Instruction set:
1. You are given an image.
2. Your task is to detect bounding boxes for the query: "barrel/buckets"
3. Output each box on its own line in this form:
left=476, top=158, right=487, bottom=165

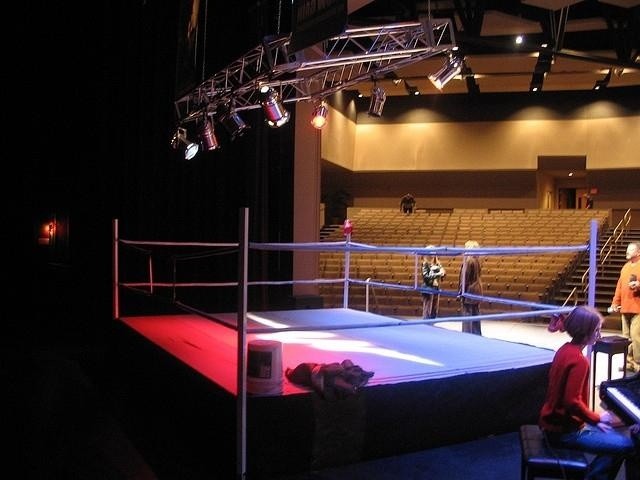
left=248, top=341, right=283, bottom=397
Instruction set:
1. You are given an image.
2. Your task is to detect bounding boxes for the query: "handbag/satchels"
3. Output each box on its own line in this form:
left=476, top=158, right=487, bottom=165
left=464, top=280, right=484, bottom=305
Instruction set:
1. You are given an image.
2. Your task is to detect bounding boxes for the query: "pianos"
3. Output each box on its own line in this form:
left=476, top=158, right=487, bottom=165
left=600, top=369, right=639, bottom=479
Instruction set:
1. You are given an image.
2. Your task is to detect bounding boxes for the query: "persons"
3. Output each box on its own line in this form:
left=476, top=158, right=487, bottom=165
left=455, top=239, right=484, bottom=337
left=536, top=305, right=635, bottom=480
left=605, top=241, right=640, bottom=374
left=399, top=193, right=417, bottom=216
left=421, top=245, right=446, bottom=320
left=583, top=193, right=594, bottom=209
